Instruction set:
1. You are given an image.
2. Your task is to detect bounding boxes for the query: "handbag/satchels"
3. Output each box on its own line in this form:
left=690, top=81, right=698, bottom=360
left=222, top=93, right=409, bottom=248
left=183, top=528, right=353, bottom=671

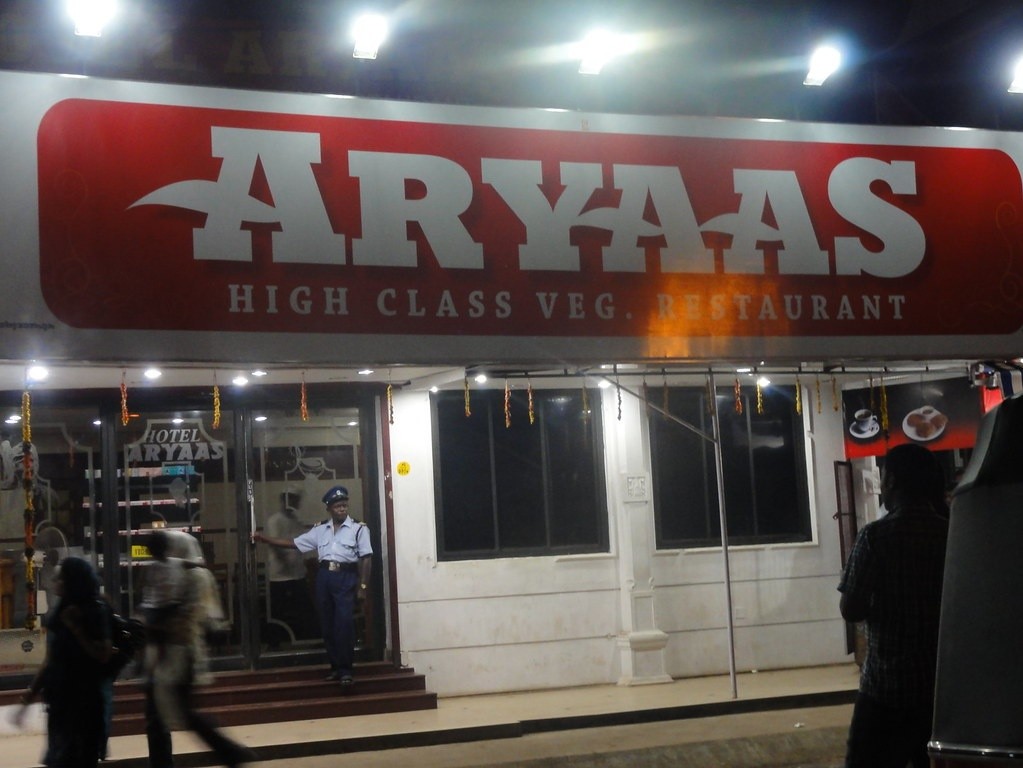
left=80, top=601, right=145, bottom=684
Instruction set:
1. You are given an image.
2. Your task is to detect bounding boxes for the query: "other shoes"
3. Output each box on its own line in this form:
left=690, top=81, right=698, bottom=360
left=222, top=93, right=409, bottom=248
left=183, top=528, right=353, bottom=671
left=326, top=673, right=338, bottom=681
left=341, top=675, right=352, bottom=684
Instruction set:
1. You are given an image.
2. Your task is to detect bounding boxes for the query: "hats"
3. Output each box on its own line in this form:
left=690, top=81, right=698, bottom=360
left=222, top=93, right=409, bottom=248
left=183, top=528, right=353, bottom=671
left=322, top=486, right=349, bottom=506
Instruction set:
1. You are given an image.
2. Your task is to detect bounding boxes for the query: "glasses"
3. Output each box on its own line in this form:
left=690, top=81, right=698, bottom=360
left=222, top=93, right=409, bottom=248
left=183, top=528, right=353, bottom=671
left=946, top=481, right=959, bottom=491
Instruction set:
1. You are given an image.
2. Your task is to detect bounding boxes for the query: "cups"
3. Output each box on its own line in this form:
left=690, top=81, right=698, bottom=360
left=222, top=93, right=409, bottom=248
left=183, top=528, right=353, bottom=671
left=855, top=410, right=876, bottom=431
left=921, top=406, right=935, bottom=419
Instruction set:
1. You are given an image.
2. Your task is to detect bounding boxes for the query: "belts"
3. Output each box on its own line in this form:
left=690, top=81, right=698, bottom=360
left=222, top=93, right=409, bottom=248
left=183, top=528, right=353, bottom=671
left=321, top=560, right=358, bottom=572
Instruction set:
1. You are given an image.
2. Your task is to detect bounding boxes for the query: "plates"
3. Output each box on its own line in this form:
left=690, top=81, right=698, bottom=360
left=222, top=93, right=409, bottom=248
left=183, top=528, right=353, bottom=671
left=902, top=408, right=944, bottom=440
left=849, top=422, right=879, bottom=439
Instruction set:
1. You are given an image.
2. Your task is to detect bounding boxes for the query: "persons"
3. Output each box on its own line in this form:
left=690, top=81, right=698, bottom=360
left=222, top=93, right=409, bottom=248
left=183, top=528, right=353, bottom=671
left=249, top=486, right=374, bottom=686
left=836, top=443, right=950, bottom=768
left=11, top=556, right=118, bottom=768
left=265, top=487, right=310, bottom=644
left=132, top=530, right=259, bottom=768
